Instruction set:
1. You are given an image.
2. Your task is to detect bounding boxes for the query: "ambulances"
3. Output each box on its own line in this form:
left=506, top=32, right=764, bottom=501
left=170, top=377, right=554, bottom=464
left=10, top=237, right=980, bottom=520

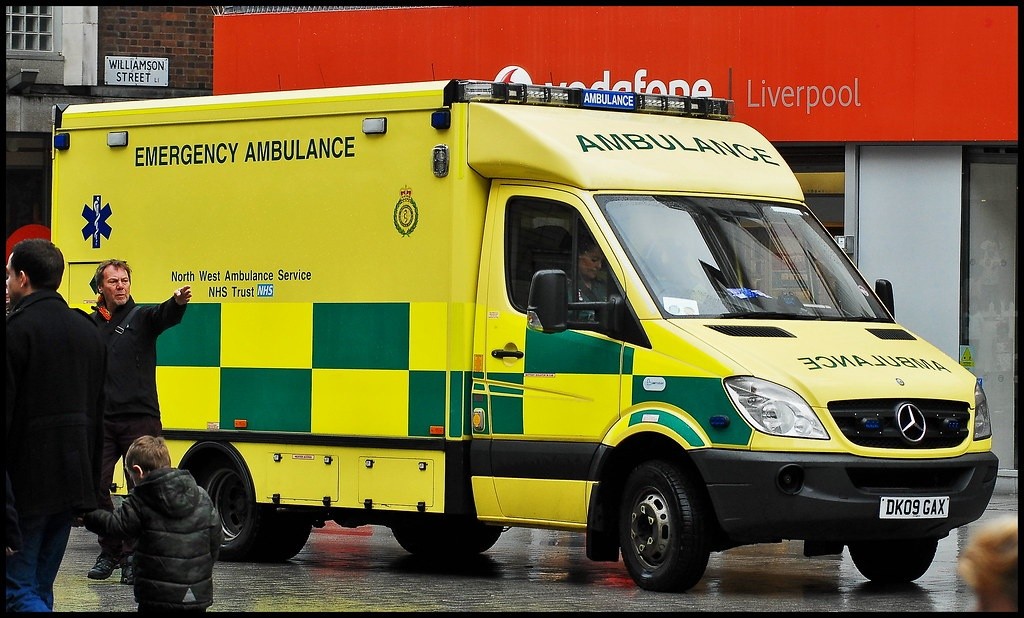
left=48, top=65, right=1001, bottom=594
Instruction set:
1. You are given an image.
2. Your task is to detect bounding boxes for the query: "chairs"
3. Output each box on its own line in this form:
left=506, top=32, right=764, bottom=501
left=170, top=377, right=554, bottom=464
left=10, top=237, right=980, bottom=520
left=517, top=225, right=572, bottom=282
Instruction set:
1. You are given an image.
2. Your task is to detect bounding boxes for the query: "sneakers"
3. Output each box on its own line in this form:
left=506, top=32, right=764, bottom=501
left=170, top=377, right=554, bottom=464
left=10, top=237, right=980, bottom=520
left=121, top=556, right=134, bottom=585
left=87, top=550, right=121, bottom=580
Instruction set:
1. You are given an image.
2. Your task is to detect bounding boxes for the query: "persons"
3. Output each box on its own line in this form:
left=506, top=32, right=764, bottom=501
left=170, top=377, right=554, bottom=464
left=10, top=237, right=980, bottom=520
left=958, top=515, right=1018, bottom=612
left=6, top=238, right=108, bottom=613
left=559, top=226, right=606, bottom=323
left=88, top=261, right=192, bottom=585
left=6, top=472, right=23, bottom=556
left=84, top=435, right=222, bottom=613
